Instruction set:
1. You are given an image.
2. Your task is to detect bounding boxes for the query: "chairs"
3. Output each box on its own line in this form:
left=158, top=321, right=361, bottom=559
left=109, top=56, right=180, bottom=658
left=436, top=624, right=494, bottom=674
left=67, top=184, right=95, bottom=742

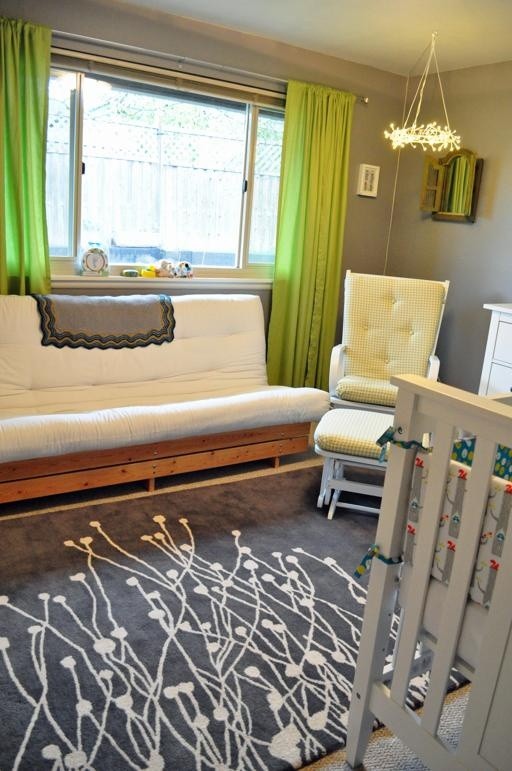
left=328, top=268, right=450, bottom=413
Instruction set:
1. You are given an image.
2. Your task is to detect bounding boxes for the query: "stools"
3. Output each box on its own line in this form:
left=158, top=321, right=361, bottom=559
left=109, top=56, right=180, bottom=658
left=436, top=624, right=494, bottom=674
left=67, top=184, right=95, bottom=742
left=312, top=408, right=430, bottom=521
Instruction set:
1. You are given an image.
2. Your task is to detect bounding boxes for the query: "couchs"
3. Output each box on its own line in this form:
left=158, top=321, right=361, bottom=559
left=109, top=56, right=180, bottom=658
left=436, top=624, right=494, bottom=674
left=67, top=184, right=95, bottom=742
left=1, top=293, right=331, bottom=507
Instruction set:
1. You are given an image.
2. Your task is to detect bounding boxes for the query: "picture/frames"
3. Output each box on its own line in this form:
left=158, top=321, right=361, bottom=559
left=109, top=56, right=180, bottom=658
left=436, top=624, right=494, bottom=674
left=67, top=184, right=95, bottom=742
left=356, top=162, right=380, bottom=197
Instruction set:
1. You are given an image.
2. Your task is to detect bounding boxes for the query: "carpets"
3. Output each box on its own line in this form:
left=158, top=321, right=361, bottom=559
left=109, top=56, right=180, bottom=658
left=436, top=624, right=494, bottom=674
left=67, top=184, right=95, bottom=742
left=0, top=461, right=472, bottom=770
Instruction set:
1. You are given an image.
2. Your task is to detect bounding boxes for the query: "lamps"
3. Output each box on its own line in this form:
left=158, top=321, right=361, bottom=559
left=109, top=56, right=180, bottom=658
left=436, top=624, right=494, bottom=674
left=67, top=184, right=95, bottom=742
left=383, top=29, right=464, bottom=154
